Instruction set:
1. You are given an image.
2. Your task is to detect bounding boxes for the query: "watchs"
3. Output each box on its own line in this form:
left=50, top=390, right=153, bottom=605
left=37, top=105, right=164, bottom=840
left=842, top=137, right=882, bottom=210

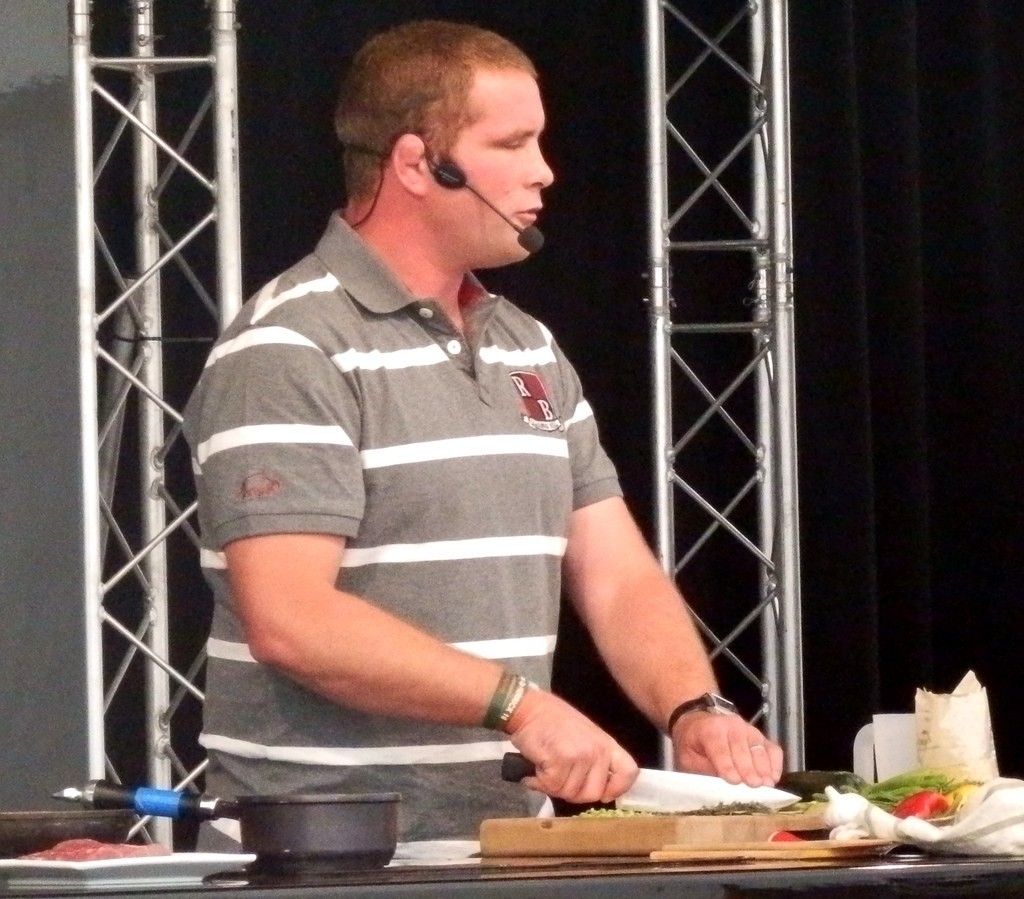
left=667, top=694, right=739, bottom=736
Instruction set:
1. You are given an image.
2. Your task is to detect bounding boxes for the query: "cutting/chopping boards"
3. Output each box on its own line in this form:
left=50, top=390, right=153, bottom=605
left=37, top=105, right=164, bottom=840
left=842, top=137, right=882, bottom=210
left=478, top=810, right=839, bottom=857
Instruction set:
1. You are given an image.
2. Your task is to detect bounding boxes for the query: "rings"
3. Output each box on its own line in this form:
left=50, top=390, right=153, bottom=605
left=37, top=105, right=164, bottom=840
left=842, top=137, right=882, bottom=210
left=750, top=744, right=764, bottom=750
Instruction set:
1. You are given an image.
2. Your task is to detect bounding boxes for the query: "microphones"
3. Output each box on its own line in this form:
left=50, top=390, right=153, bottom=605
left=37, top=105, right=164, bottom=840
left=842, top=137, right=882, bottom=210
left=432, top=163, right=545, bottom=253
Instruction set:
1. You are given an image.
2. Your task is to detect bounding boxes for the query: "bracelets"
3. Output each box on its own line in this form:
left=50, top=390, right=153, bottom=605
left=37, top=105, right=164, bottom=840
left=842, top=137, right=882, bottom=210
left=483, top=671, right=539, bottom=733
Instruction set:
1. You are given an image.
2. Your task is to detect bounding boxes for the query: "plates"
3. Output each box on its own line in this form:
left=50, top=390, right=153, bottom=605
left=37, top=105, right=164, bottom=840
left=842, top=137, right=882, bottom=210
left=0, top=852, right=258, bottom=892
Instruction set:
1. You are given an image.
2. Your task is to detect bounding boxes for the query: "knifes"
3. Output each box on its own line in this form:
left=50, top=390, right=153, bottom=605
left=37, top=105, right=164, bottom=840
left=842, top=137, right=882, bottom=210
left=501, top=753, right=802, bottom=809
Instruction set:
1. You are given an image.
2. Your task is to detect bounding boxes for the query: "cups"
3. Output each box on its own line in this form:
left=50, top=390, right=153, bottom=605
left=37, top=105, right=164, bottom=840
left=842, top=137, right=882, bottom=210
left=851, top=713, right=918, bottom=786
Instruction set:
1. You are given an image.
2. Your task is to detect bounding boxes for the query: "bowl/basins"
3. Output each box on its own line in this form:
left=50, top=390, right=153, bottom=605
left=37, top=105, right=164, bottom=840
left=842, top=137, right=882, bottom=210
left=0, top=809, right=139, bottom=855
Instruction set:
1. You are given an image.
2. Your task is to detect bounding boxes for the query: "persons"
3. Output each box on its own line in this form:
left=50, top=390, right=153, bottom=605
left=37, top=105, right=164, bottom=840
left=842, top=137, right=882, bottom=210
left=182, top=21, right=786, bottom=853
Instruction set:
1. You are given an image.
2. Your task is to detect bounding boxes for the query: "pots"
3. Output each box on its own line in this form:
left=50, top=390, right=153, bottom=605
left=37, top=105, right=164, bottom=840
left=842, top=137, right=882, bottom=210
left=49, top=779, right=403, bottom=872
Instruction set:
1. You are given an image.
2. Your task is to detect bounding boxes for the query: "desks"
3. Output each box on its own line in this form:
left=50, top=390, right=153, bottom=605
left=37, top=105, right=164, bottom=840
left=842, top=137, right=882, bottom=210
left=0, top=850, right=1024, bottom=899
